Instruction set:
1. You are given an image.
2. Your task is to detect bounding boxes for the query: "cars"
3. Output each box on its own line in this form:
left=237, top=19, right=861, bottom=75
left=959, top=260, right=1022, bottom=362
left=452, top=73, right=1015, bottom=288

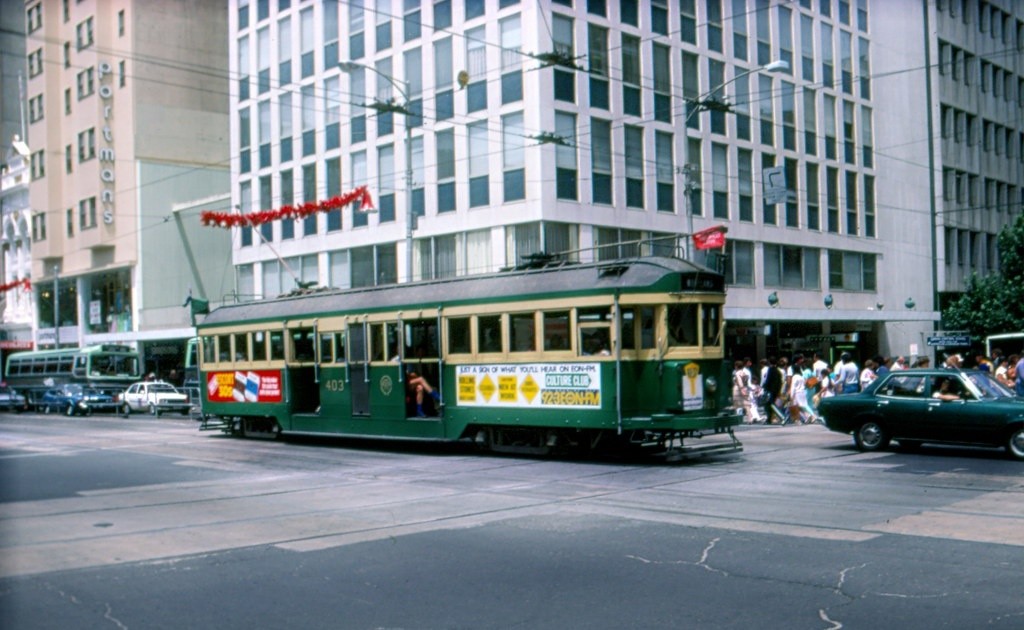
left=115, top=382, right=192, bottom=416
left=0, top=383, right=26, bottom=414
left=34, top=383, right=117, bottom=414
left=817, top=368, right=1024, bottom=463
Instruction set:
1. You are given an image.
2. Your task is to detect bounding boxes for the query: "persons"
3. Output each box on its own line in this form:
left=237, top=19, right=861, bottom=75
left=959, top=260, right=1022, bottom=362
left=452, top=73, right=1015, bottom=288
left=732, top=348, right=1024, bottom=425
left=485, top=329, right=502, bottom=352
left=391, top=345, right=440, bottom=418
left=582, top=332, right=610, bottom=356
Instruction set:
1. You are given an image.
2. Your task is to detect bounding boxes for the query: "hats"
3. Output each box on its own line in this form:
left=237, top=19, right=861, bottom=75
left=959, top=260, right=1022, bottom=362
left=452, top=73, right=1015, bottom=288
left=991, top=349, right=1002, bottom=356
left=917, top=356, right=929, bottom=366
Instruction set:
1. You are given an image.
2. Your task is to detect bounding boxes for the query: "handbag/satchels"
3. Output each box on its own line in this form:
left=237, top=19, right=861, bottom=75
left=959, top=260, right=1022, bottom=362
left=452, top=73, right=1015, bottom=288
left=787, top=399, right=799, bottom=421
left=734, top=375, right=749, bottom=387
left=807, top=376, right=818, bottom=389
left=757, top=388, right=770, bottom=407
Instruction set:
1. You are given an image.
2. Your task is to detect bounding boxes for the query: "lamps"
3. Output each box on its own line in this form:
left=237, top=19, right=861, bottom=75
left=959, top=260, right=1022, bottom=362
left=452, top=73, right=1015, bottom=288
left=768, top=292, right=778, bottom=308
left=906, top=297, right=915, bottom=311
left=824, top=294, right=833, bottom=309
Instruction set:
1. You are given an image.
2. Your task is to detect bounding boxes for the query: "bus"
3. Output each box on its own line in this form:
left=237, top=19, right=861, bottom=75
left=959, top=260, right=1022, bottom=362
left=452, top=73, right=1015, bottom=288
left=184, top=337, right=207, bottom=399
left=4, top=344, right=142, bottom=397
left=181, top=224, right=745, bottom=463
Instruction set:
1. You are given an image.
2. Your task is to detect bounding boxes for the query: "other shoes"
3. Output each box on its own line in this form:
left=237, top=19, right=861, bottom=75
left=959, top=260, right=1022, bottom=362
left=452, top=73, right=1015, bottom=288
left=804, top=413, right=811, bottom=424
left=811, top=415, right=818, bottom=425
left=763, top=419, right=771, bottom=425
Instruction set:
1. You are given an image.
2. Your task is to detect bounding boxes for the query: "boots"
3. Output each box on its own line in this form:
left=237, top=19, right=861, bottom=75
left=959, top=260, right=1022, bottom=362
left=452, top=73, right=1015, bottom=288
left=429, top=391, right=440, bottom=402
left=417, top=404, right=426, bottom=418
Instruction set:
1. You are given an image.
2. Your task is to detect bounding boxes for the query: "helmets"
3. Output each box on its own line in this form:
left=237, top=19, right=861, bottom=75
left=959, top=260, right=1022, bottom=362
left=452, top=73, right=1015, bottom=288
left=841, top=351, right=852, bottom=363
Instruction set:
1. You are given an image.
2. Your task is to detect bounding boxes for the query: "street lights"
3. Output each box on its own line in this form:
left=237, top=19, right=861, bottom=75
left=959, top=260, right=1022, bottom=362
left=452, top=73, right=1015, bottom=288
left=336, top=60, right=413, bottom=284
left=683, top=60, right=790, bottom=265
